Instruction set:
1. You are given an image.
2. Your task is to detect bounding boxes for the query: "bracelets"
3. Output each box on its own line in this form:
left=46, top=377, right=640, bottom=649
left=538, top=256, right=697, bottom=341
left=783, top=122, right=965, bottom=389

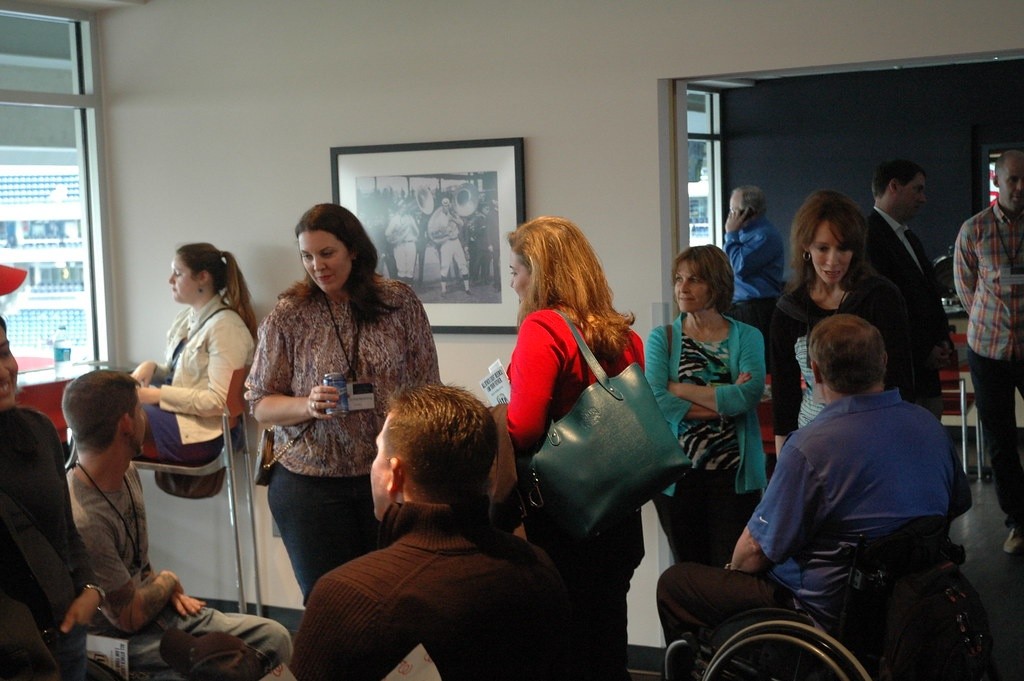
left=85, top=584, right=106, bottom=607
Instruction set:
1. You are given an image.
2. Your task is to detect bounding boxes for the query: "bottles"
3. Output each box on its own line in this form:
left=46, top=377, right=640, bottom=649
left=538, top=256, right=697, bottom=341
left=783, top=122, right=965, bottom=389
left=54, top=324, right=71, bottom=380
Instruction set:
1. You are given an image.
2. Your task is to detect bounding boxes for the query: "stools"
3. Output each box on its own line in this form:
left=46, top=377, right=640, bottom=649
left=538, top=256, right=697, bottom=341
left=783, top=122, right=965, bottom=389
left=133, top=363, right=267, bottom=615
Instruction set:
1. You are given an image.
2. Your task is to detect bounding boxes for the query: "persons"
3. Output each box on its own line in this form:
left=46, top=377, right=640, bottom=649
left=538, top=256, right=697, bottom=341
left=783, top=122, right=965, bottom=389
left=953, top=150, right=1024, bottom=553
left=644, top=160, right=955, bottom=566
left=499, top=215, right=692, bottom=681
left=245, top=204, right=440, bottom=605
left=0, top=312, right=123, bottom=681
left=281, top=384, right=568, bottom=681
left=61, top=370, right=294, bottom=680
left=655, top=316, right=1000, bottom=681
left=125, top=243, right=262, bottom=464
left=355, top=175, right=500, bottom=299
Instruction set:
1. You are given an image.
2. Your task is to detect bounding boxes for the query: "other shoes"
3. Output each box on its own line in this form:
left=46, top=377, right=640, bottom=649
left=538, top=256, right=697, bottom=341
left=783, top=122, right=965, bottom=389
left=1004, top=527, right=1024, bottom=554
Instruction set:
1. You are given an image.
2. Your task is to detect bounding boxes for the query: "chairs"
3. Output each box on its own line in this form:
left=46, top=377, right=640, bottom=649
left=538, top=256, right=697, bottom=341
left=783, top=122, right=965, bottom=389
left=938, top=349, right=981, bottom=485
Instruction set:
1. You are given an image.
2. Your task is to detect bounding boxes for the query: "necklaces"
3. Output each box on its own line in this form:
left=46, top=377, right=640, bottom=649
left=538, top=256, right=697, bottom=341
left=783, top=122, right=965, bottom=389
left=683, top=320, right=724, bottom=348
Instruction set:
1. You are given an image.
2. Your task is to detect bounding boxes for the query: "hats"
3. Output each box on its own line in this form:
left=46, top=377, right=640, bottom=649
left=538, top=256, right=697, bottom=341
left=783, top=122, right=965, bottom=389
left=160, top=628, right=264, bottom=681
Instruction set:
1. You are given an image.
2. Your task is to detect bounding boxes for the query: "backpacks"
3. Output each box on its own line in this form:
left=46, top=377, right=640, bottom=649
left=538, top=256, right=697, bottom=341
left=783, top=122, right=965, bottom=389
left=878, top=562, right=994, bottom=681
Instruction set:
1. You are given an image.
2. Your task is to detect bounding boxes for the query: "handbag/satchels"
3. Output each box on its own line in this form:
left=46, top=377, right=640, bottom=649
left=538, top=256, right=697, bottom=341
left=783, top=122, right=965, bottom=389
left=153, top=464, right=226, bottom=500
left=516, top=309, right=692, bottom=543
left=253, top=428, right=275, bottom=485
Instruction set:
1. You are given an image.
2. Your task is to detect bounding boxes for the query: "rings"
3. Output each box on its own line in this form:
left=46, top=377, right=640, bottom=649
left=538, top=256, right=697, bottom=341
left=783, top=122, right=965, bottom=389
left=311, top=402, right=316, bottom=409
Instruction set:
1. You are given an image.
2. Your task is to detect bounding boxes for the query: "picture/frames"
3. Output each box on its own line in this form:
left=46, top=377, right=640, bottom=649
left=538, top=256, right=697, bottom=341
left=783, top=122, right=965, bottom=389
left=329, top=138, right=531, bottom=335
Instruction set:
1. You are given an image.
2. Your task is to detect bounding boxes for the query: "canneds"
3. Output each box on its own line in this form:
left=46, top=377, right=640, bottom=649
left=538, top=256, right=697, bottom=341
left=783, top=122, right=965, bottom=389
left=323, top=372, right=349, bottom=416
